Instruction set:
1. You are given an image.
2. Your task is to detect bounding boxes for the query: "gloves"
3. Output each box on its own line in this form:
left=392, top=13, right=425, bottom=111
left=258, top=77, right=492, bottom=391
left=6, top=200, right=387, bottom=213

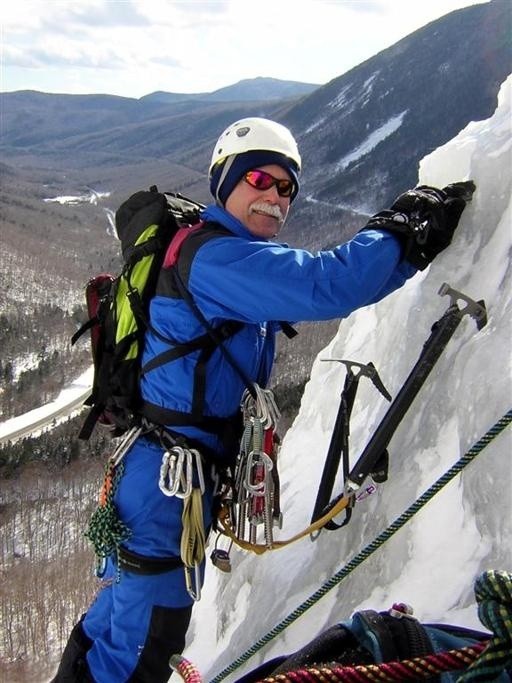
left=358, top=179, right=476, bottom=271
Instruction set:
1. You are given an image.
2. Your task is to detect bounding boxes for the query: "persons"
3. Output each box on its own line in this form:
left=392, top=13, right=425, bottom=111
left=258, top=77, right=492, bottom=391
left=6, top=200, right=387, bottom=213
left=52, top=118, right=472, bottom=682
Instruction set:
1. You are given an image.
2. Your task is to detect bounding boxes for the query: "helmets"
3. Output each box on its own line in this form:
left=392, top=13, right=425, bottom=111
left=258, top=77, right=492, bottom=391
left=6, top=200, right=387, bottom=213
left=208, top=117, right=302, bottom=178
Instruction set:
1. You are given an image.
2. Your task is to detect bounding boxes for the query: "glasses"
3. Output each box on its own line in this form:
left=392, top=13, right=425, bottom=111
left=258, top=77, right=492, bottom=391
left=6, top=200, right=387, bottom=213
left=239, top=166, right=294, bottom=199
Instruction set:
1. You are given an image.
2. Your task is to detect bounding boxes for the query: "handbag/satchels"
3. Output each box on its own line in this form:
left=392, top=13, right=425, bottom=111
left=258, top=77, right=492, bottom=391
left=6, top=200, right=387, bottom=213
left=259, top=602, right=490, bottom=683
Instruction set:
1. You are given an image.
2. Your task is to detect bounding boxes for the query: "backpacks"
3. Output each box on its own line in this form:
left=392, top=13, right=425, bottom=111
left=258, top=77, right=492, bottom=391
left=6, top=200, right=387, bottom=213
left=85, top=184, right=238, bottom=429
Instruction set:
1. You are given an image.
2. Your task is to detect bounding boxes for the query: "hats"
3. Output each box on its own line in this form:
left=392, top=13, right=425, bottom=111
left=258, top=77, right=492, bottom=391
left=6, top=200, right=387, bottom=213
left=209, top=151, right=300, bottom=210
